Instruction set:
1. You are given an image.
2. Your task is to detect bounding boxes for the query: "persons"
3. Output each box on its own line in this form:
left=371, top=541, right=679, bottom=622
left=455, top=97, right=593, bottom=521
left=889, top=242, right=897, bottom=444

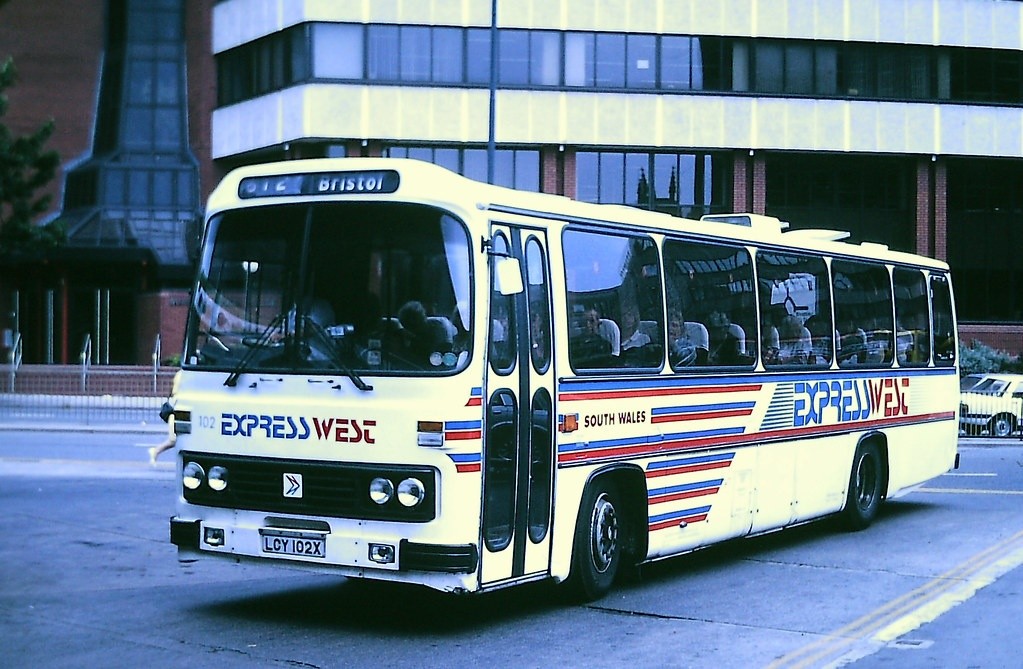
left=612, top=303, right=652, bottom=352
left=569, top=305, right=614, bottom=368
left=702, top=311, right=909, bottom=366
left=269, top=279, right=337, bottom=359
left=667, top=308, right=697, bottom=367
left=396, top=300, right=447, bottom=355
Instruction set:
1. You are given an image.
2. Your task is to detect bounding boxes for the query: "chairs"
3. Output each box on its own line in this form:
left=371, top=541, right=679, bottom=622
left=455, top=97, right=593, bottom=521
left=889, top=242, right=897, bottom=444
left=368, top=308, right=938, bottom=375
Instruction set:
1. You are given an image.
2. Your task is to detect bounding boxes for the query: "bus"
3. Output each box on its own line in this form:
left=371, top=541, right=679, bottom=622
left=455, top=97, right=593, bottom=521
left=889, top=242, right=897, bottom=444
left=168, top=158, right=962, bottom=602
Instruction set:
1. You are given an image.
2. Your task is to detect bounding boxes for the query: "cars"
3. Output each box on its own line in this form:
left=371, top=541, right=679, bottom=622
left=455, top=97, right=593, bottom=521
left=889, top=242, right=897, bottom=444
left=962, top=373, right=1023, bottom=437
